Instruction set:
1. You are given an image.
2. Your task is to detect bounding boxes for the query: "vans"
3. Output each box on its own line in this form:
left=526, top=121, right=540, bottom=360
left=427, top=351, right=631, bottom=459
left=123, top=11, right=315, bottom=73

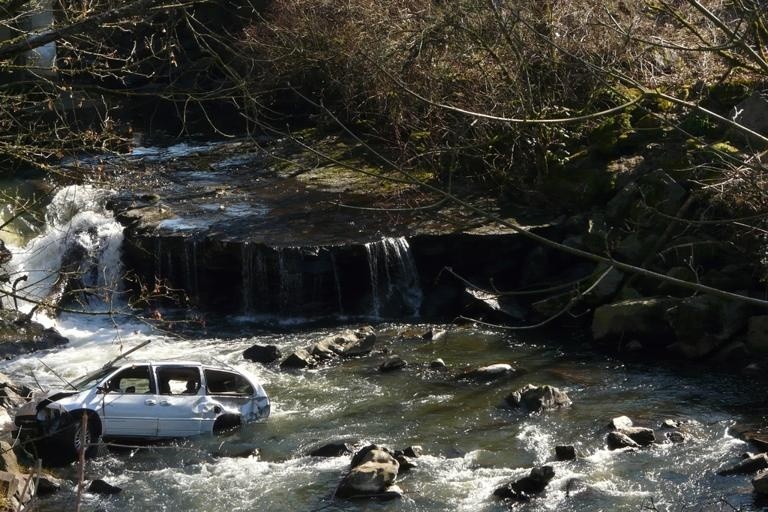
left=16, top=361, right=272, bottom=459
